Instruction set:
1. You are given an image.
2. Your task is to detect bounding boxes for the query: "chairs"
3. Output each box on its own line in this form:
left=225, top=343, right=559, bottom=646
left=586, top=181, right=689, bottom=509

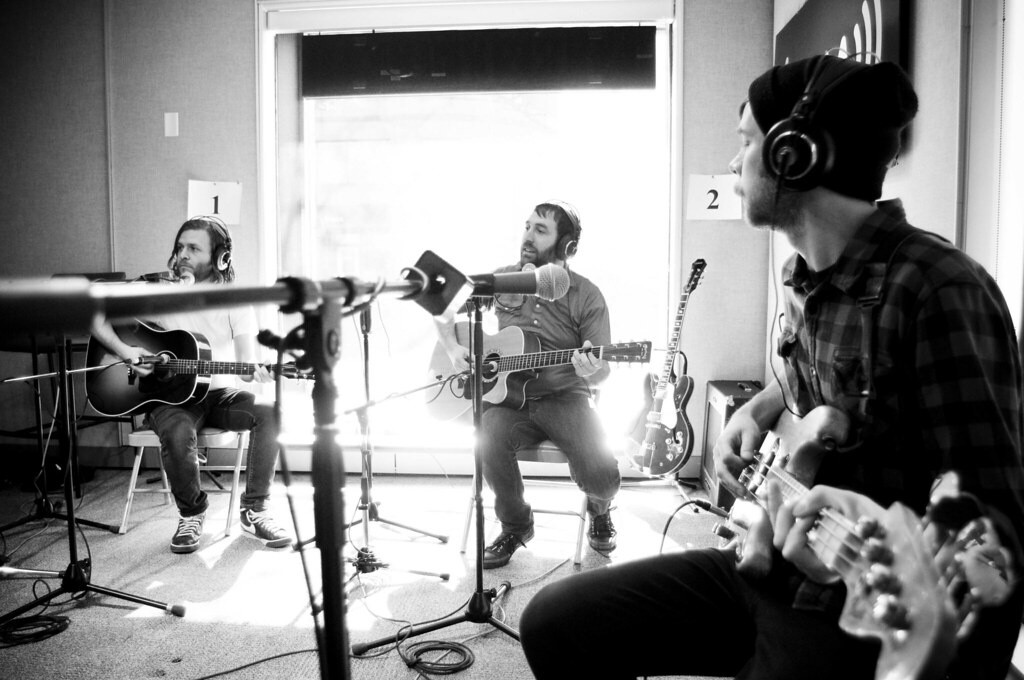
left=118, top=423, right=249, bottom=537
left=457, top=388, right=601, bottom=565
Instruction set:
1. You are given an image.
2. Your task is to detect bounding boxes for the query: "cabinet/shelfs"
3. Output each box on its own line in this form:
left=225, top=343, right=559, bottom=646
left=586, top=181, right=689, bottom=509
left=0, top=339, right=142, bottom=499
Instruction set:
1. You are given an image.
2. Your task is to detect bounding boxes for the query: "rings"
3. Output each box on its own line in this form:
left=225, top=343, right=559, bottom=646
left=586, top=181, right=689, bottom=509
left=580, top=364, right=583, bottom=367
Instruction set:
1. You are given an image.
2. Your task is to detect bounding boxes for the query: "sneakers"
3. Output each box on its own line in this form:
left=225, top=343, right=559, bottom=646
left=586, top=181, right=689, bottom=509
left=587, top=506, right=618, bottom=550
left=483, top=525, right=535, bottom=569
left=239, top=506, right=292, bottom=548
left=171, top=511, right=206, bottom=552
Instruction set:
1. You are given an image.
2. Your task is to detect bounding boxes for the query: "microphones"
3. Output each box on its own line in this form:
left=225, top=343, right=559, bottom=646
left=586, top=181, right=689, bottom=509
left=463, top=262, right=570, bottom=302
left=462, top=360, right=497, bottom=375
left=178, top=272, right=195, bottom=286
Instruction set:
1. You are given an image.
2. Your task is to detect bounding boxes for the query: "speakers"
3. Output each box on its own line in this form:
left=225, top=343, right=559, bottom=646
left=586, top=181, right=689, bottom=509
left=701, top=381, right=768, bottom=508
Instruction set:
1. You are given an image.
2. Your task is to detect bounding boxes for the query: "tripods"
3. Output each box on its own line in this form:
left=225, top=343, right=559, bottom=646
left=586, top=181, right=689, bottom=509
left=0, top=332, right=186, bottom=623
left=293, top=278, right=523, bottom=658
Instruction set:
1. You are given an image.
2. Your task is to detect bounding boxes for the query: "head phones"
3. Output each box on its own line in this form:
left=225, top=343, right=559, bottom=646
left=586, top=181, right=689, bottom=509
left=763, top=48, right=881, bottom=191
left=545, top=201, right=580, bottom=261
left=187, top=215, right=232, bottom=271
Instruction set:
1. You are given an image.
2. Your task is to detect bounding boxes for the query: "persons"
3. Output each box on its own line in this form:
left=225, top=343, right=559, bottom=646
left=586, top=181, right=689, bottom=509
left=89, top=219, right=292, bottom=556
left=519, top=53, right=1024, bottom=680
left=434, top=202, right=623, bottom=570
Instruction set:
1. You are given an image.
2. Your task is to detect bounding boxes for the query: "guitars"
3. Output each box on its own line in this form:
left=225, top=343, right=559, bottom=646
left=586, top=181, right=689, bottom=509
left=623, top=258, right=707, bottom=479
left=83, top=317, right=315, bottom=417
left=713, top=404, right=1014, bottom=680
left=425, top=322, right=652, bottom=426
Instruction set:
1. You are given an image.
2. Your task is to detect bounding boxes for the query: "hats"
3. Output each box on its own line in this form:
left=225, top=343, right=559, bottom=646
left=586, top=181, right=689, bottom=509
left=749, top=54, right=919, bottom=202
